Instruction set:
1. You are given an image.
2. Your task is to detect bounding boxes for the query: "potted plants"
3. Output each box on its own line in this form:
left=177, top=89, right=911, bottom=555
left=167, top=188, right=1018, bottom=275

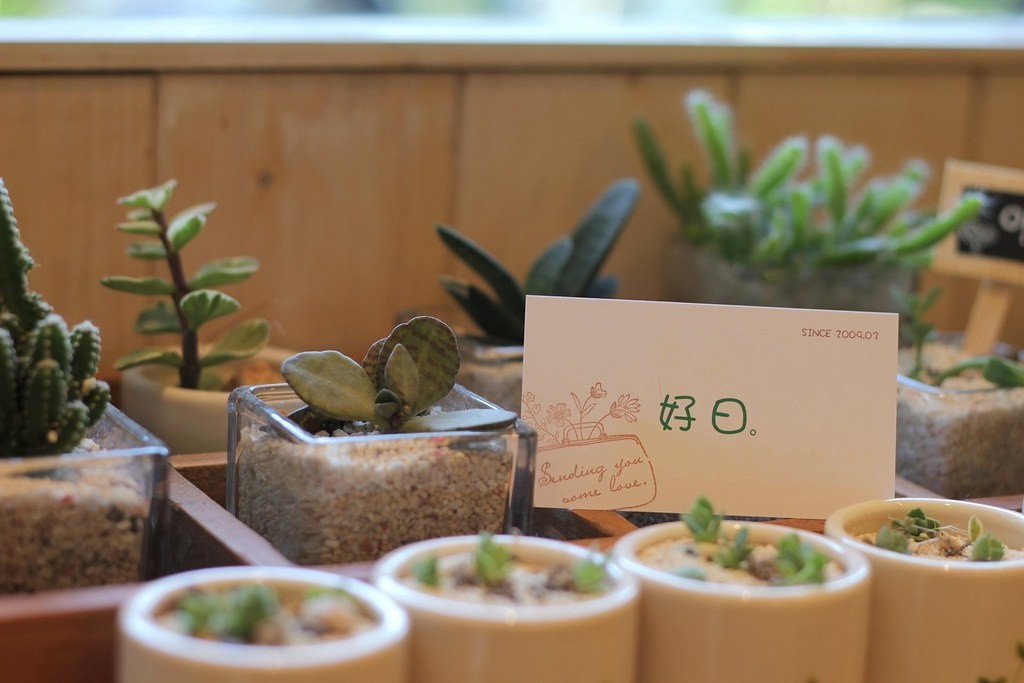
left=0, top=86, right=1024, bottom=682
